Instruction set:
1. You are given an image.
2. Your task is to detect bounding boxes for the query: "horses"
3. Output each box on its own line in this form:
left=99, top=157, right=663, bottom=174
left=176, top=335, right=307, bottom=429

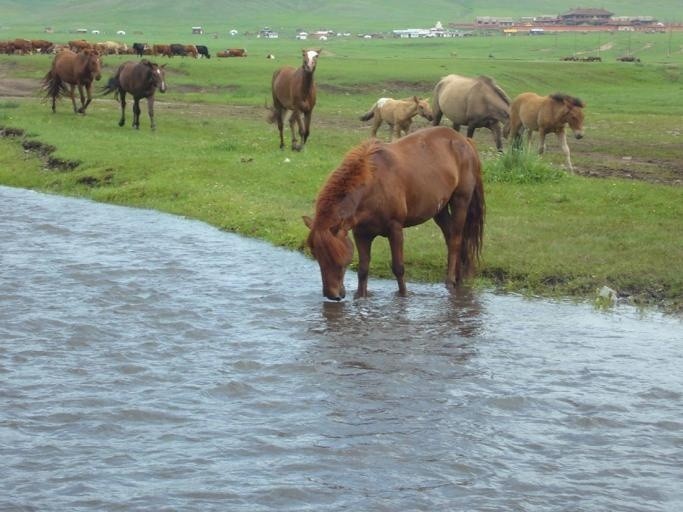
left=99, top=59, right=167, bottom=131
left=40, top=49, right=102, bottom=114
left=359, top=95, right=433, bottom=140
left=301, top=125, right=487, bottom=301
left=217, top=48, right=248, bottom=57
left=265, top=48, right=323, bottom=152
left=432, top=74, right=524, bottom=155
left=0, top=39, right=213, bottom=56
left=509, top=92, right=585, bottom=173
